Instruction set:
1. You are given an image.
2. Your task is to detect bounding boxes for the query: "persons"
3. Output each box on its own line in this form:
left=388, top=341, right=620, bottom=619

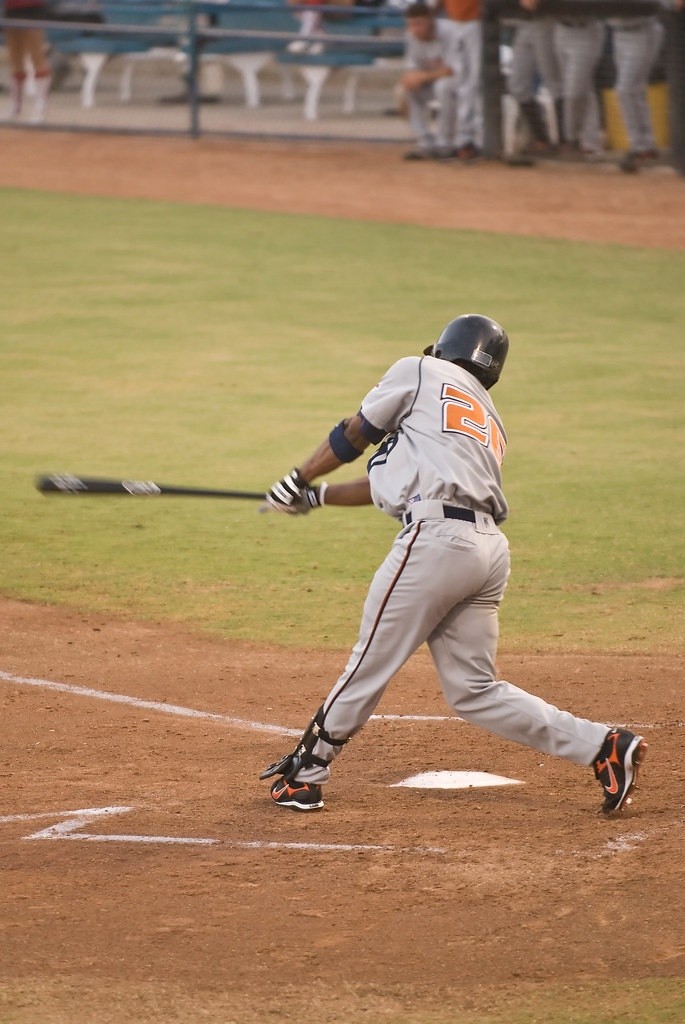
left=158, top=0, right=229, bottom=104
left=265, top=312, right=649, bottom=816
left=285, top=0, right=354, bottom=54
left=4, top=0, right=55, bottom=121
left=50, top=0, right=107, bottom=80
left=381, top=0, right=685, bottom=175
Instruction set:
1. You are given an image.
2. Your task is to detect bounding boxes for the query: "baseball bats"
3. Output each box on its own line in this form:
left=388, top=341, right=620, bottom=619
left=34, top=471, right=269, bottom=501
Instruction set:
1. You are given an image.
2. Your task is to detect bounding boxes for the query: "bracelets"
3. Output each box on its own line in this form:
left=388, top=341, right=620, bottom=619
left=319, top=482, right=327, bottom=507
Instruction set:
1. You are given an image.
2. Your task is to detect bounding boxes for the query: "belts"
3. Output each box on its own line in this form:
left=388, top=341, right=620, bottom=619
left=405, top=504, right=476, bottom=523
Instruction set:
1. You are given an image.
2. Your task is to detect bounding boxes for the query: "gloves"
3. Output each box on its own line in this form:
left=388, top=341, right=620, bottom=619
left=264, top=468, right=327, bottom=515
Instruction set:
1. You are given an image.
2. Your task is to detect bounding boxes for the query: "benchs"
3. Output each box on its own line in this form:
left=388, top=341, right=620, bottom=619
left=0, top=0, right=618, bottom=145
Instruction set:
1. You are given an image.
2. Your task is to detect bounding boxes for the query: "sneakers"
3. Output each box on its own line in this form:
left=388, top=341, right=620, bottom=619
left=593, top=728, right=647, bottom=815
left=270, top=776, right=324, bottom=812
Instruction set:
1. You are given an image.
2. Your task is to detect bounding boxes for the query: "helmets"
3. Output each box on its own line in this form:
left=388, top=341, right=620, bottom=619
left=423, top=314, right=509, bottom=390
